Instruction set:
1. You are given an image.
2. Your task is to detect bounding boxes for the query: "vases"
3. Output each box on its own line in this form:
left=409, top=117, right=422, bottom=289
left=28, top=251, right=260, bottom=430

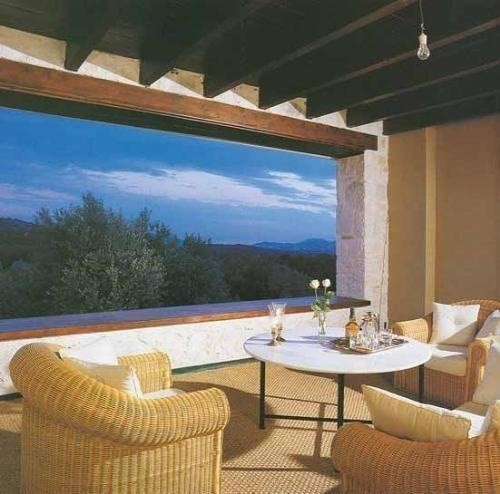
left=309, top=278, right=335, bottom=335
left=316, top=309, right=329, bottom=337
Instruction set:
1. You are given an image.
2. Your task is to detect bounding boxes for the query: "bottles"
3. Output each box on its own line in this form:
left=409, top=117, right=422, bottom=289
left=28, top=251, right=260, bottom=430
left=347, top=305, right=360, bottom=343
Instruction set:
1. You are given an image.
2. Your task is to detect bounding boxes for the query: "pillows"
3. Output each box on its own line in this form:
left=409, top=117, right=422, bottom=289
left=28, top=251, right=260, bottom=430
left=56, top=335, right=142, bottom=397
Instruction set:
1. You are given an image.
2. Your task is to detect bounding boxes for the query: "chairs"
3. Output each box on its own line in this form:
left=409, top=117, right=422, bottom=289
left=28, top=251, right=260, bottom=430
left=7, top=339, right=234, bottom=494
left=328, top=298, right=500, bottom=494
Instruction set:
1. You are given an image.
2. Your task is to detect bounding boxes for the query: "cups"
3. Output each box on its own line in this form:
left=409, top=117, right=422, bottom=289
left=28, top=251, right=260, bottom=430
left=350, top=322, right=392, bottom=352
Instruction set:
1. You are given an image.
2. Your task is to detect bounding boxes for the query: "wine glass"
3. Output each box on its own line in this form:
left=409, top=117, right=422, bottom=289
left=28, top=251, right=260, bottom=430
left=268, top=302, right=286, bottom=346
